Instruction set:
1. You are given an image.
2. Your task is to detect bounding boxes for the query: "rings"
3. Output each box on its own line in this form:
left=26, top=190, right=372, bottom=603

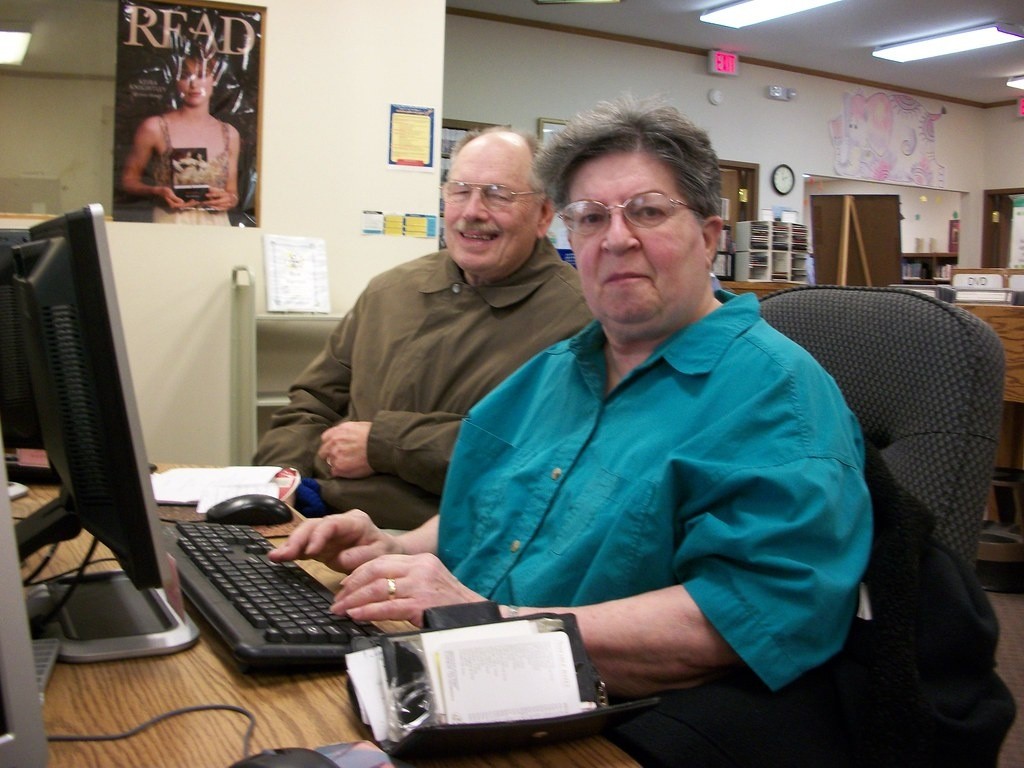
left=386, top=577, right=396, bottom=600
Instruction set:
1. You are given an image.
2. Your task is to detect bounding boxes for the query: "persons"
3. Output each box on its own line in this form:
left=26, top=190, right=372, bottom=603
left=269, top=94, right=871, bottom=768
left=251, top=126, right=593, bottom=531
left=123, top=45, right=239, bottom=228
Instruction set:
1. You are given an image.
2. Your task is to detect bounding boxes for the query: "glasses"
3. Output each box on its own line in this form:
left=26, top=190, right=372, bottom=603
left=557, top=189, right=704, bottom=238
left=439, top=180, right=542, bottom=212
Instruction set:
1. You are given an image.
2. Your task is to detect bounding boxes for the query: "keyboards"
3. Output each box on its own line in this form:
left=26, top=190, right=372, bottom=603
left=162, top=521, right=390, bottom=675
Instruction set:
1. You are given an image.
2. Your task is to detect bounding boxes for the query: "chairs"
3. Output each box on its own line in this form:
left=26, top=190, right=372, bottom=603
left=716, top=286, right=1008, bottom=559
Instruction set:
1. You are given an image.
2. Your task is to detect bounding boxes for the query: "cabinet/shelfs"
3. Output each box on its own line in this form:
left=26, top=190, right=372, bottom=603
left=732, top=221, right=809, bottom=287
left=899, top=252, right=959, bottom=286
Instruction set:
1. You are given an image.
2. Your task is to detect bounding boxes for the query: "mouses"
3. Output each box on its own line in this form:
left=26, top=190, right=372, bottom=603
left=228, top=747, right=341, bottom=768
left=207, top=494, right=293, bottom=525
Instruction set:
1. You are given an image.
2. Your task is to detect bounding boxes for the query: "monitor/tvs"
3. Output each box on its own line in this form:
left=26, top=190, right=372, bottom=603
left=0, top=202, right=200, bottom=661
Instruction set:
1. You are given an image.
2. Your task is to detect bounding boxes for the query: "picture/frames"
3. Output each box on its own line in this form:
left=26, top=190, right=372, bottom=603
left=539, top=119, right=571, bottom=147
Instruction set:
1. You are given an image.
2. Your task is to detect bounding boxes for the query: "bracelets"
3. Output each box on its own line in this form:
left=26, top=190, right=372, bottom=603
left=508, top=605, right=517, bottom=618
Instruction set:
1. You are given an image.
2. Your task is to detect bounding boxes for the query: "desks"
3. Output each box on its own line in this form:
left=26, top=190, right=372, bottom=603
left=7, top=465, right=644, bottom=766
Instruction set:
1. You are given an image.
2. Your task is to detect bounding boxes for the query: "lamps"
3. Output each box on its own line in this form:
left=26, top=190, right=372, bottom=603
left=739, top=188, right=747, bottom=202
left=766, top=85, right=797, bottom=101
left=992, top=210, right=1000, bottom=222
left=871, top=21, right=1023, bottom=62
left=1007, top=76, right=1024, bottom=89
left=698, top=0, right=856, bottom=29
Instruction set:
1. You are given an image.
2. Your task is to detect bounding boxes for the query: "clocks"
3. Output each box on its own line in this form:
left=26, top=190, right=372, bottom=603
left=773, top=165, right=795, bottom=193
